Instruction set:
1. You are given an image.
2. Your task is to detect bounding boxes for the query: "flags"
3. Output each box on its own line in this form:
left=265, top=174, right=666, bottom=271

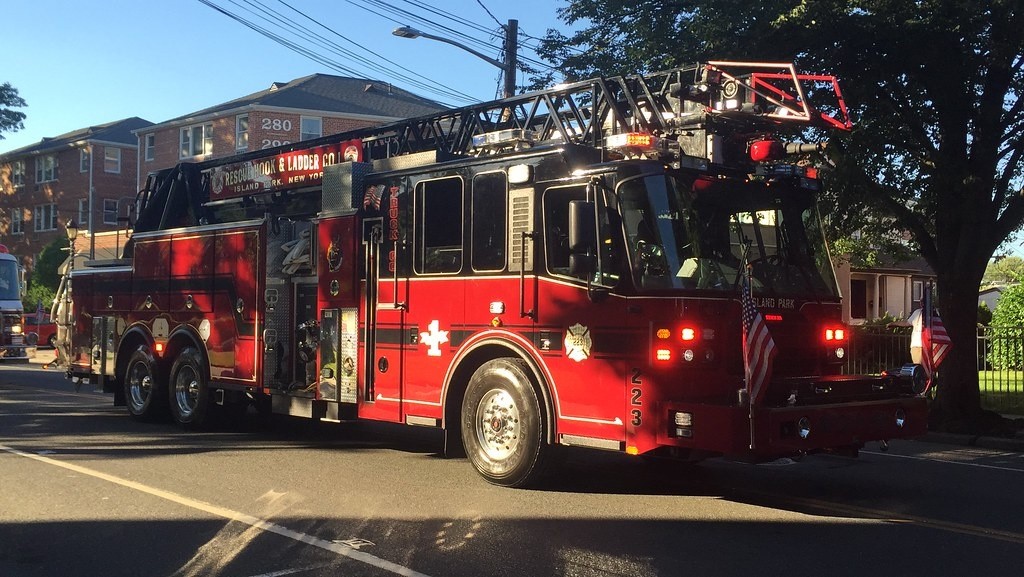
left=741, top=280, right=778, bottom=408
left=36, top=301, right=46, bottom=321
left=921, top=307, right=954, bottom=394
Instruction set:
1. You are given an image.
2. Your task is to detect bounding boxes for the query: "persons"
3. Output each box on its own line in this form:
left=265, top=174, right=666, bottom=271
left=886, top=297, right=921, bottom=364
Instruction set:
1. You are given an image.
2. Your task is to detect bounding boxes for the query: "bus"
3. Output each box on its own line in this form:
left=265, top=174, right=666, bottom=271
left=0, top=244, right=38, bottom=366
left=65, top=60, right=933, bottom=488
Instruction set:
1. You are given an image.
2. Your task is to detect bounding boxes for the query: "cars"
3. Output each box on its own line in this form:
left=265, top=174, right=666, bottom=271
left=21, top=312, right=58, bottom=351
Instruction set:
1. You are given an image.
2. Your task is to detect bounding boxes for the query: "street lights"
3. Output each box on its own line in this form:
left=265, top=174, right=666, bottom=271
left=393, top=25, right=516, bottom=123
left=65, top=217, right=80, bottom=357
